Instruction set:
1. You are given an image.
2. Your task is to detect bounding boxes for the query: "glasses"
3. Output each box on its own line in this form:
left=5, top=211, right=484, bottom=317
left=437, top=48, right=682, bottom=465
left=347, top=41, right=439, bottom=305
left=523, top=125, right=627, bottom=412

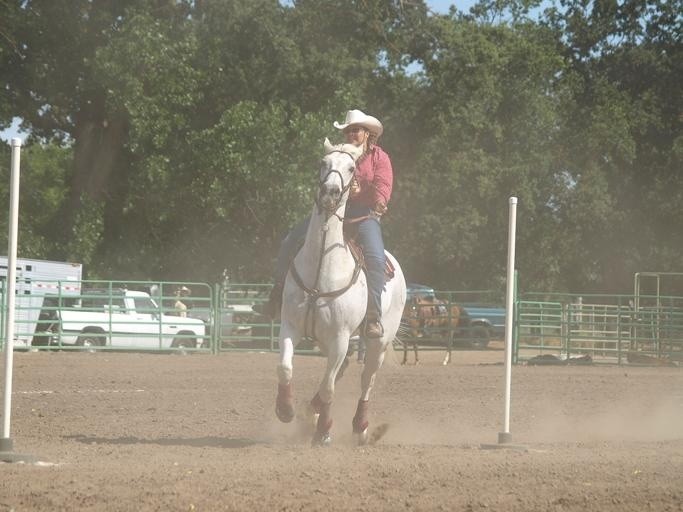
left=343, top=127, right=359, bottom=134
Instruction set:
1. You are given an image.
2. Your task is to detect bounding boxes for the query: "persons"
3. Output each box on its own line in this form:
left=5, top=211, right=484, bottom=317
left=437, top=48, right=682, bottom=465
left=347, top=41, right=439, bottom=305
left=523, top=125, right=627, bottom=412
left=251, top=109, right=393, bottom=337
left=180, top=286, right=195, bottom=308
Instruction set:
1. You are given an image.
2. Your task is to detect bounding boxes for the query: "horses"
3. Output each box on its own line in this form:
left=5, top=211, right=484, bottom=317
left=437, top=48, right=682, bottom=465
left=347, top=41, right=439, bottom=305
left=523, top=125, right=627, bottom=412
left=276, top=137, right=408, bottom=447
left=402, top=298, right=464, bottom=366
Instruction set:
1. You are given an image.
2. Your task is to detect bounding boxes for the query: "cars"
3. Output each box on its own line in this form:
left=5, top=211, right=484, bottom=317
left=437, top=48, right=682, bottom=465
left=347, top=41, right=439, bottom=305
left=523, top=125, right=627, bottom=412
left=191, top=286, right=261, bottom=347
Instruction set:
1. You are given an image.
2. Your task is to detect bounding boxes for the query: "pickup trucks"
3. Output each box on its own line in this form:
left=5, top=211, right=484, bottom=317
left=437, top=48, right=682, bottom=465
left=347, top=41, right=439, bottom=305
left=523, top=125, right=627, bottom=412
left=401, top=277, right=505, bottom=348
left=45, top=288, right=204, bottom=352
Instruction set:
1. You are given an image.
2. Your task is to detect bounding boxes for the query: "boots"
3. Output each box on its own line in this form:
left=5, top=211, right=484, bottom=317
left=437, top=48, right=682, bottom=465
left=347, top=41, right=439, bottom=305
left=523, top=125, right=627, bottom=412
left=366, top=319, right=383, bottom=339
left=252, top=278, right=284, bottom=319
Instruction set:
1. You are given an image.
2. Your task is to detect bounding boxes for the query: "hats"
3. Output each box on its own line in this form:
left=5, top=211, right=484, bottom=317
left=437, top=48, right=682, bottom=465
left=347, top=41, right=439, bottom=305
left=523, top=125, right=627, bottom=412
left=181, top=287, right=191, bottom=297
left=333, top=109, right=383, bottom=136
